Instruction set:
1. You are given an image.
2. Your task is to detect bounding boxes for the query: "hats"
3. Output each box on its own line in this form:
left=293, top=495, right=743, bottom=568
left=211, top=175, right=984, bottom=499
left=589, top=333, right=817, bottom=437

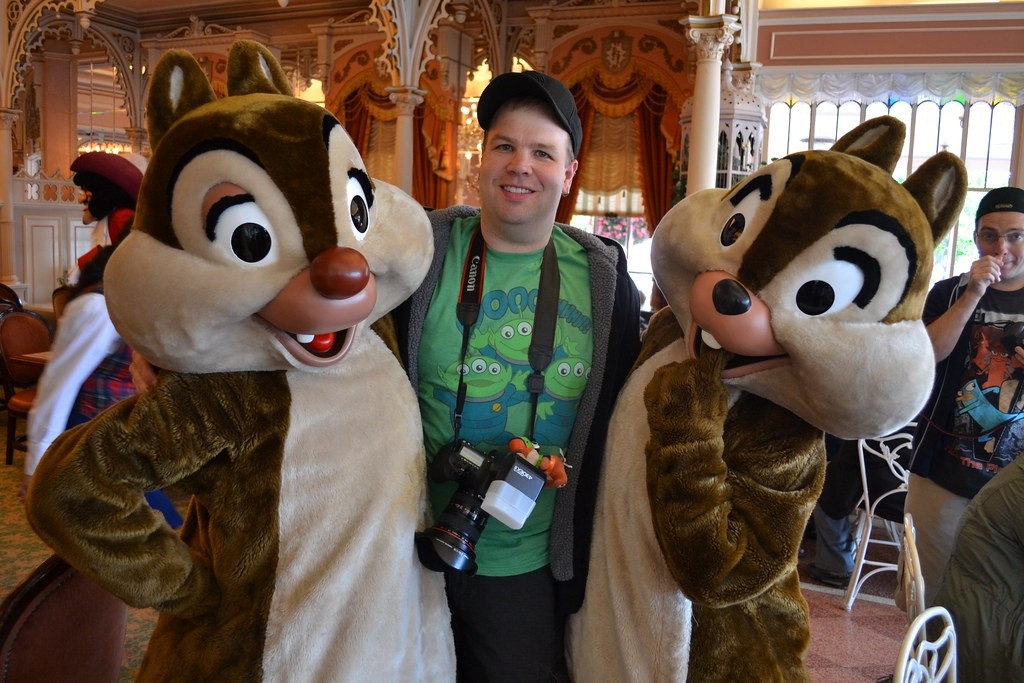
left=975, top=187, right=1024, bottom=223
left=68, top=151, right=146, bottom=202
left=477, top=70, right=582, bottom=158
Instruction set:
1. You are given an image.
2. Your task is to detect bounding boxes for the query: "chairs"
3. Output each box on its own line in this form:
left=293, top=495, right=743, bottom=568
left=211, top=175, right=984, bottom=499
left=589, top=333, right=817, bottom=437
left=842, top=421, right=957, bottom=683
left=0, top=554, right=127, bottom=683
left=0, top=282, right=72, bottom=465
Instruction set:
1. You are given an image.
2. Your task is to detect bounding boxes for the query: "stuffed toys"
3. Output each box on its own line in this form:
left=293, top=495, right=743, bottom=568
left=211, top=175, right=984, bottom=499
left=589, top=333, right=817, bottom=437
left=561, top=115, right=969, bottom=683
left=23, top=38, right=459, bottom=683
left=62, top=153, right=144, bottom=292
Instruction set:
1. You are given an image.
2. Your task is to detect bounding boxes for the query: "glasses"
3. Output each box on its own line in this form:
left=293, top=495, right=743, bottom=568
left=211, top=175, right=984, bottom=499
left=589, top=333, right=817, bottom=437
left=975, top=231, right=1024, bottom=244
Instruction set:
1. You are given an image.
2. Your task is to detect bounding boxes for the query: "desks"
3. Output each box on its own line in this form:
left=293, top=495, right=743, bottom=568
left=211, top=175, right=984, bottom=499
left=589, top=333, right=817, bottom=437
left=9, top=352, right=52, bottom=367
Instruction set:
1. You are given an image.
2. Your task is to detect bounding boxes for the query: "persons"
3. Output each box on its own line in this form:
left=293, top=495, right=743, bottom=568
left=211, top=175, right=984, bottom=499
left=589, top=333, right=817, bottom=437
left=367, top=71, right=642, bottom=683
left=24, top=216, right=185, bottom=538
left=877, top=188, right=1024, bottom=683
left=928, top=449, right=1024, bottom=683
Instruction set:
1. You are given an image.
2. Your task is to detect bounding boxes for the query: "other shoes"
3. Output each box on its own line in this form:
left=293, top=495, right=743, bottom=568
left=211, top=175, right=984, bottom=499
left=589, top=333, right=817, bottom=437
left=798, top=546, right=806, bottom=558
left=805, top=562, right=851, bottom=590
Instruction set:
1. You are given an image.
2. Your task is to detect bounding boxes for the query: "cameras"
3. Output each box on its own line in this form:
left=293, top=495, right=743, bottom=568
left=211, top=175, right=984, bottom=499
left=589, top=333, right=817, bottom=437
left=416, top=439, right=547, bottom=577
left=1001, top=322, right=1024, bottom=355
